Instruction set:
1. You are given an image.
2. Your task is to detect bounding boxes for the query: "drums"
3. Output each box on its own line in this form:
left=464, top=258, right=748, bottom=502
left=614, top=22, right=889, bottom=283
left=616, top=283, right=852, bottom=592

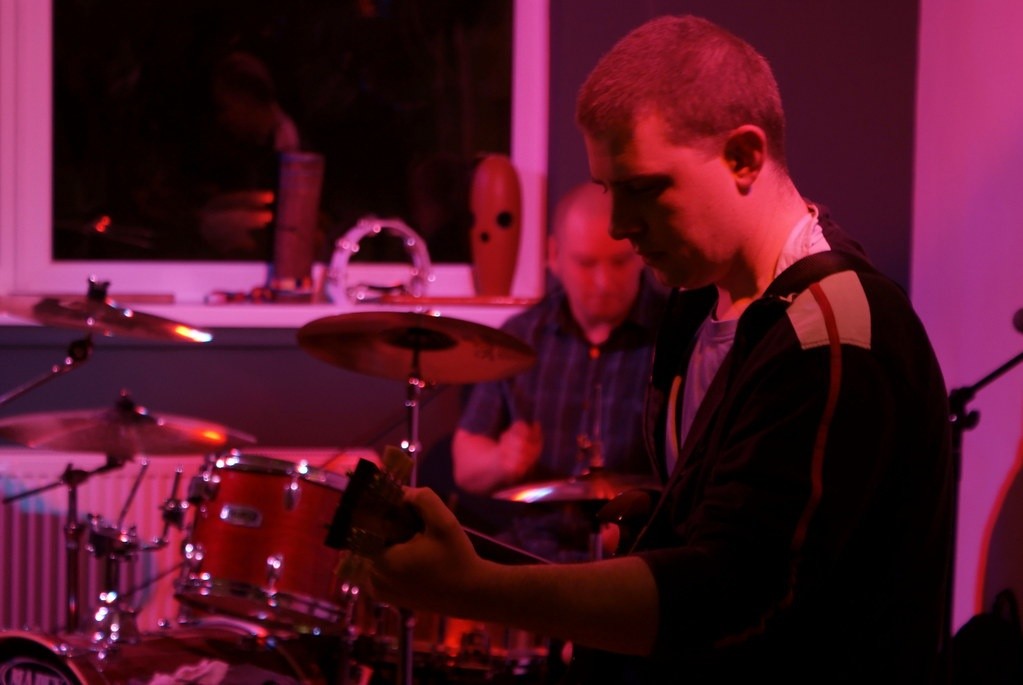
left=1, top=613, right=315, bottom=685
left=173, top=451, right=364, bottom=639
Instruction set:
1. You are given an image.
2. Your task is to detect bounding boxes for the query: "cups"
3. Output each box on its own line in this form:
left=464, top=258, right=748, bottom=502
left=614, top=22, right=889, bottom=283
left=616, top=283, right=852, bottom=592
left=267, top=151, right=324, bottom=302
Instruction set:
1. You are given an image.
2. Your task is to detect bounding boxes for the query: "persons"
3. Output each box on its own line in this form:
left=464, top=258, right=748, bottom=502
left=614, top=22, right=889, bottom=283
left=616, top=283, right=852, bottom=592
left=352, top=14, right=953, bottom=685
left=452, top=186, right=667, bottom=563
left=209, top=51, right=301, bottom=260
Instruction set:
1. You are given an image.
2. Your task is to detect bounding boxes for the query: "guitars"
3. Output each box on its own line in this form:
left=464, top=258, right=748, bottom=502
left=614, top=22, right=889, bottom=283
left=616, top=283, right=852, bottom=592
left=318, top=452, right=552, bottom=570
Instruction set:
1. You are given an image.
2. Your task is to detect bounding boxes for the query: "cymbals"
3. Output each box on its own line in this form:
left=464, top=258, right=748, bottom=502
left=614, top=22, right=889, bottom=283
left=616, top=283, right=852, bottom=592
left=1, top=273, right=217, bottom=345
left=325, top=212, right=438, bottom=306
left=1, top=386, right=258, bottom=459
left=496, top=468, right=661, bottom=508
left=293, top=306, right=541, bottom=390
